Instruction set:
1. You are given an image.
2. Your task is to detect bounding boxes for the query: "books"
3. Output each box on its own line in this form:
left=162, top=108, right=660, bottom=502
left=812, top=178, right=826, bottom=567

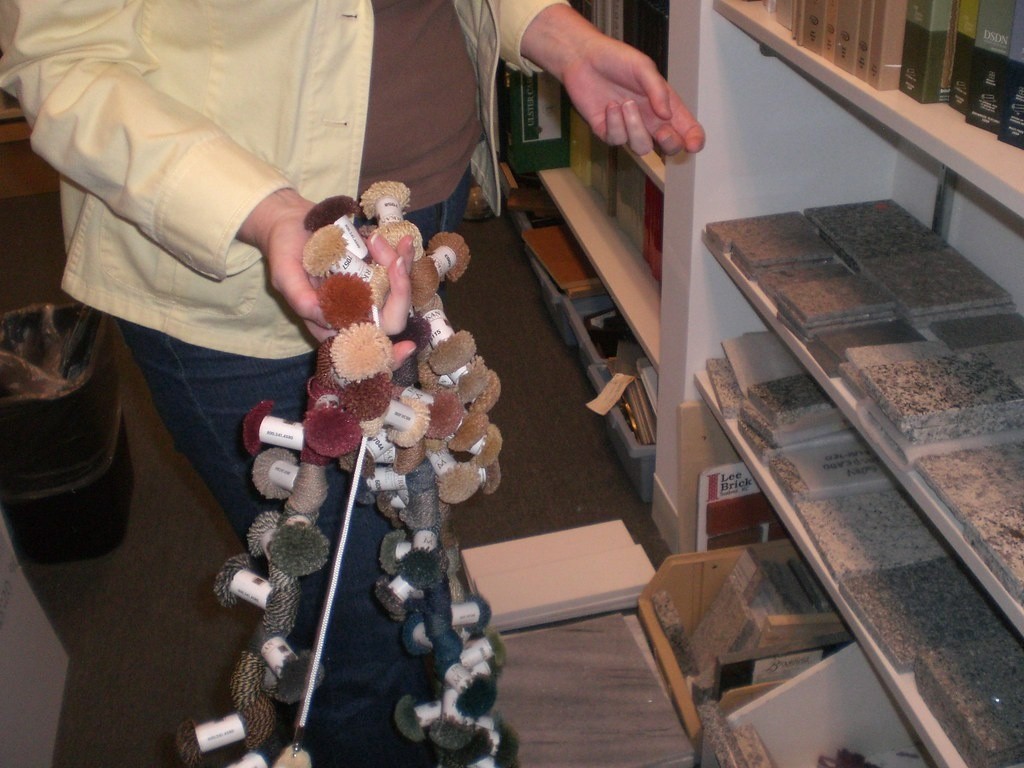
left=753, top=2, right=1024, bottom=149
left=498, top=0, right=670, bottom=446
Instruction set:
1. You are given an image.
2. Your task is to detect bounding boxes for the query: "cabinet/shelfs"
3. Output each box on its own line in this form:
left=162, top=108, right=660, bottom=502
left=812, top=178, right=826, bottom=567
left=466, top=0, right=1024, bottom=768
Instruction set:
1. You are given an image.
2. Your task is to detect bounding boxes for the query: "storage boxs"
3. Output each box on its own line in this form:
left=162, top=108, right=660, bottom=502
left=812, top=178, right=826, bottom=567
left=636, top=540, right=856, bottom=741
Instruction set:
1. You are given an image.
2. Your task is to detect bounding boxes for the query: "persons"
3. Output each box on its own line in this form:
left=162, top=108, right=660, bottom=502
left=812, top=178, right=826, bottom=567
left=0, top=1, right=706, bottom=767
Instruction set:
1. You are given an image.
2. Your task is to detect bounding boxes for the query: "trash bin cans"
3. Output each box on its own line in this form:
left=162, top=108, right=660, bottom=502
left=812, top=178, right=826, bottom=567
left=0, top=302, right=135, bottom=565
